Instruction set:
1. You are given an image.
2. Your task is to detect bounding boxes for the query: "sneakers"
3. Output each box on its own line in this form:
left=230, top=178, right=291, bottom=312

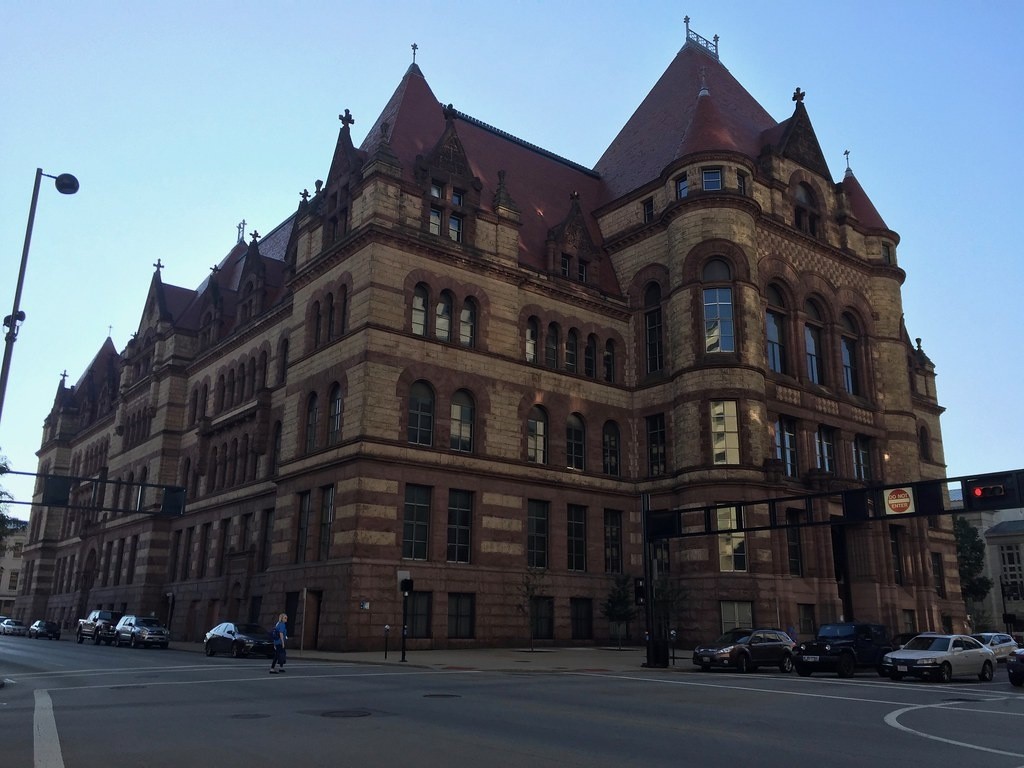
left=269, top=670, right=279, bottom=674
left=279, top=669, right=286, bottom=673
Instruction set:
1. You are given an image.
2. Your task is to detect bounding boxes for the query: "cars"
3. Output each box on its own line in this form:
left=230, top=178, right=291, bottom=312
left=1006, top=649, right=1024, bottom=688
left=114, top=615, right=170, bottom=649
left=968, top=632, right=1017, bottom=663
left=0, top=619, right=26, bottom=636
left=204, top=622, right=274, bottom=658
left=883, top=633, right=998, bottom=683
left=28, top=620, right=60, bottom=640
left=692, top=626, right=796, bottom=675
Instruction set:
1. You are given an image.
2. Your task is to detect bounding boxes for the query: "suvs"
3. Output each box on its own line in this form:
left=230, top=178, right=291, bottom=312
left=793, top=621, right=892, bottom=676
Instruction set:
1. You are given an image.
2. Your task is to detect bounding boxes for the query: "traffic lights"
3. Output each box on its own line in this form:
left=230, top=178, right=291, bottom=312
left=969, top=484, right=1004, bottom=499
left=401, top=578, right=413, bottom=592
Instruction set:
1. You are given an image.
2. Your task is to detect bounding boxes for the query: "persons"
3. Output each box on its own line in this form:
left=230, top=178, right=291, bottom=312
left=269, top=613, right=289, bottom=675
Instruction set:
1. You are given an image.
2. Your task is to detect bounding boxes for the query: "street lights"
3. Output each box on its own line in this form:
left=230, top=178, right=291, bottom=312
left=0, top=166, right=77, bottom=424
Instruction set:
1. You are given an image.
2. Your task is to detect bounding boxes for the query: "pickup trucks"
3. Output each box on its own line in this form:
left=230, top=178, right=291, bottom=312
left=76, top=609, right=126, bottom=644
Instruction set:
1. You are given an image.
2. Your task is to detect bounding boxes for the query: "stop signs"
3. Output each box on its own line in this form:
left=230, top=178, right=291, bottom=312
left=886, top=488, right=912, bottom=512
